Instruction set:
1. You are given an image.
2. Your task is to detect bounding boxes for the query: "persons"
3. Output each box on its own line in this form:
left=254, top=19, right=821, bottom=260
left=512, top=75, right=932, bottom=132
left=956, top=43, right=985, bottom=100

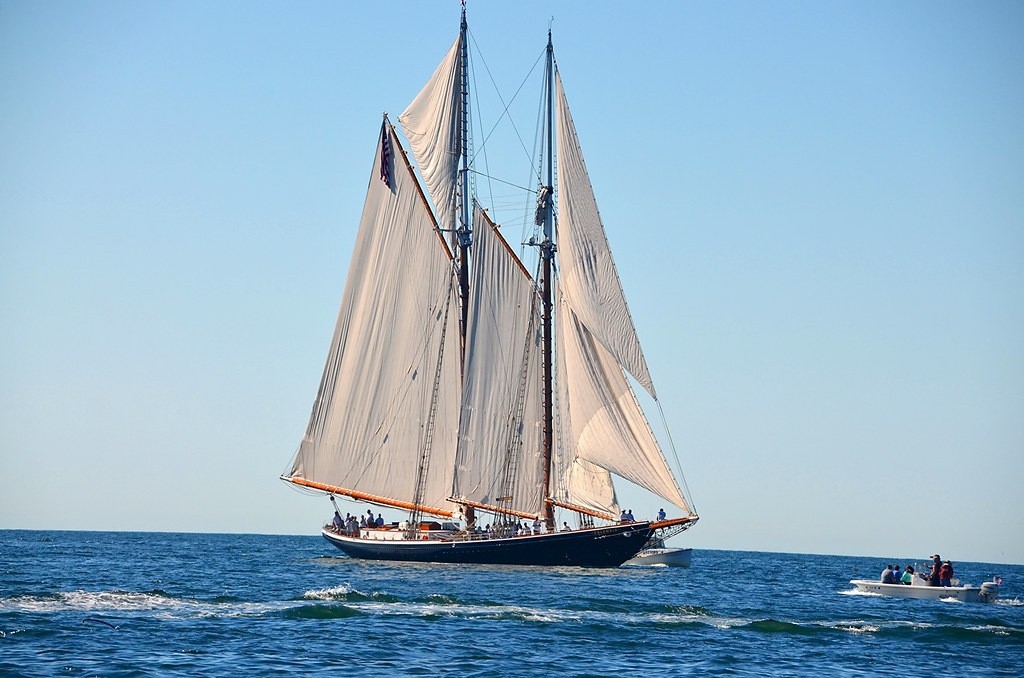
left=621, top=509, right=634, bottom=522
left=402, top=520, right=410, bottom=531
left=476, top=526, right=482, bottom=533
left=514, top=522, right=531, bottom=536
left=563, top=522, right=570, bottom=530
left=881, top=564, right=914, bottom=585
left=332, top=511, right=359, bottom=538
left=657, top=509, right=671, bottom=533
left=924, top=555, right=954, bottom=587
left=533, top=516, right=542, bottom=534
left=359, top=509, right=384, bottom=528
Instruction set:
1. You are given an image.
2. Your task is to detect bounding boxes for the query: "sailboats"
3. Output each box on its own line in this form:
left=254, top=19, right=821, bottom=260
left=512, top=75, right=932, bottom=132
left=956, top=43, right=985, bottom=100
left=279, top=0, right=701, bottom=570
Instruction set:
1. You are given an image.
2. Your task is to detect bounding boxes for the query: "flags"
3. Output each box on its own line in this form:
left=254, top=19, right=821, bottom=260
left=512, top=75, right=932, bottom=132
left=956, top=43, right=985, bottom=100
left=380, top=122, right=397, bottom=194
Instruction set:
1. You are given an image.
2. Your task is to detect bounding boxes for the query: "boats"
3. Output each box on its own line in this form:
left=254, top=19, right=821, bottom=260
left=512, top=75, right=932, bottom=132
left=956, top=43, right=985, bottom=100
left=622, top=535, right=694, bottom=568
left=849, top=575, right=1002, bottom=605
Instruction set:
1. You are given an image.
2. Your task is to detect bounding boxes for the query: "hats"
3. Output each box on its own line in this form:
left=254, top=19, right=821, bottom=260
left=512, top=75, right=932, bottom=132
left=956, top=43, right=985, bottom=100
left=944, top=559, right=951, bottom=563
left=930, top=554, right=940, bottom=559
left=887, top=565, right=894, bottom=568
